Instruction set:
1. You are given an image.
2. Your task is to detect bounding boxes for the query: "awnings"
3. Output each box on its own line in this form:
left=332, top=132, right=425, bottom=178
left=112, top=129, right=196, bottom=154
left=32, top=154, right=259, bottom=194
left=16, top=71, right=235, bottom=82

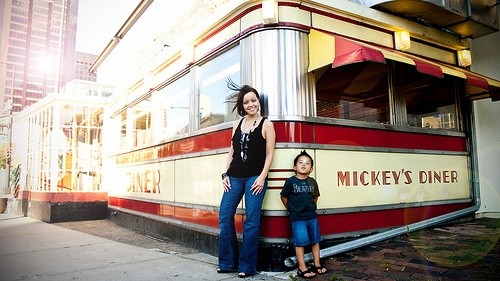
left=308, top=29, right=500, bottom=100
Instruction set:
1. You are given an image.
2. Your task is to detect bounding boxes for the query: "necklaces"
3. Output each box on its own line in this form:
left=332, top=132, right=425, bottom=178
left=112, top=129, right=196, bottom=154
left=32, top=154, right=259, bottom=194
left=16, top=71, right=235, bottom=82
left=240, top=114, right=259, bottom=163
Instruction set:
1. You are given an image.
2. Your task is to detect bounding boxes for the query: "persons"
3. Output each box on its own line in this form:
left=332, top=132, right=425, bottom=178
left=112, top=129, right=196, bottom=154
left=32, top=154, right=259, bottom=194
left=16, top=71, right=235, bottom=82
left=216, top=76, right=276, bottom=278
left=279, top=149, right=327, bottom=278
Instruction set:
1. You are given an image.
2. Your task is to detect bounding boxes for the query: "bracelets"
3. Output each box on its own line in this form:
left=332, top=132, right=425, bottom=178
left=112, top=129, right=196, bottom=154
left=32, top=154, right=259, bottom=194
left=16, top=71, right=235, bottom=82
left=222, top=173, right=228, bottom=180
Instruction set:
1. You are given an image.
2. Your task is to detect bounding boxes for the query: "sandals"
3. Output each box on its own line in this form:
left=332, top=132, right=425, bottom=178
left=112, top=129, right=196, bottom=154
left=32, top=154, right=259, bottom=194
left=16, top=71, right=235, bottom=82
left=297, top=264, right=327, bottom=278
left=217, top=266, right=248, bottom=278
